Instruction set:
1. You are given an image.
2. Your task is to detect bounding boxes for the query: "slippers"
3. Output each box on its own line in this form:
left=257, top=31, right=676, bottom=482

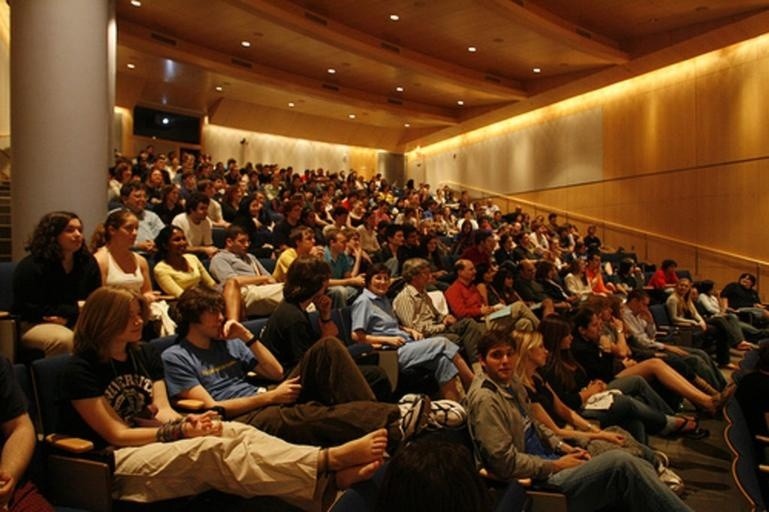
left=685, top=428, right=709, bottom=440
left=673, top=413, right=699, bottom=436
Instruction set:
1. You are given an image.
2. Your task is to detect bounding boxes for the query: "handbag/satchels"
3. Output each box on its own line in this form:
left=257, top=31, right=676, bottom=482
left=654, top=451, right=685, bottom=497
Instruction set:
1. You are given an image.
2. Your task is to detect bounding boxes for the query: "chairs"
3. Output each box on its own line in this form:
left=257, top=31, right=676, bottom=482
left=131, top=225, right=353, bottom=364
left=721, top=336, right=767, bottom=512
left=1, top=225, right=110, bottom=512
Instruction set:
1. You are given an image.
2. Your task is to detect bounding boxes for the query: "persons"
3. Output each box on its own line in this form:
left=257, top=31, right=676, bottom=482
left=4, top=145, right=767, bottom=510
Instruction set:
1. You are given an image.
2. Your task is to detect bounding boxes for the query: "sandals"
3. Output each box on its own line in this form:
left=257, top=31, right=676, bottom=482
left=711, top=382, right=736, bottom=418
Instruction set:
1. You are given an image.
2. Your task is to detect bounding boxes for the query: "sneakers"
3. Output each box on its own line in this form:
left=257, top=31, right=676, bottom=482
left=386, top=394, right=432, bottom=456
left=396, top=393, right=468, bottom=432
